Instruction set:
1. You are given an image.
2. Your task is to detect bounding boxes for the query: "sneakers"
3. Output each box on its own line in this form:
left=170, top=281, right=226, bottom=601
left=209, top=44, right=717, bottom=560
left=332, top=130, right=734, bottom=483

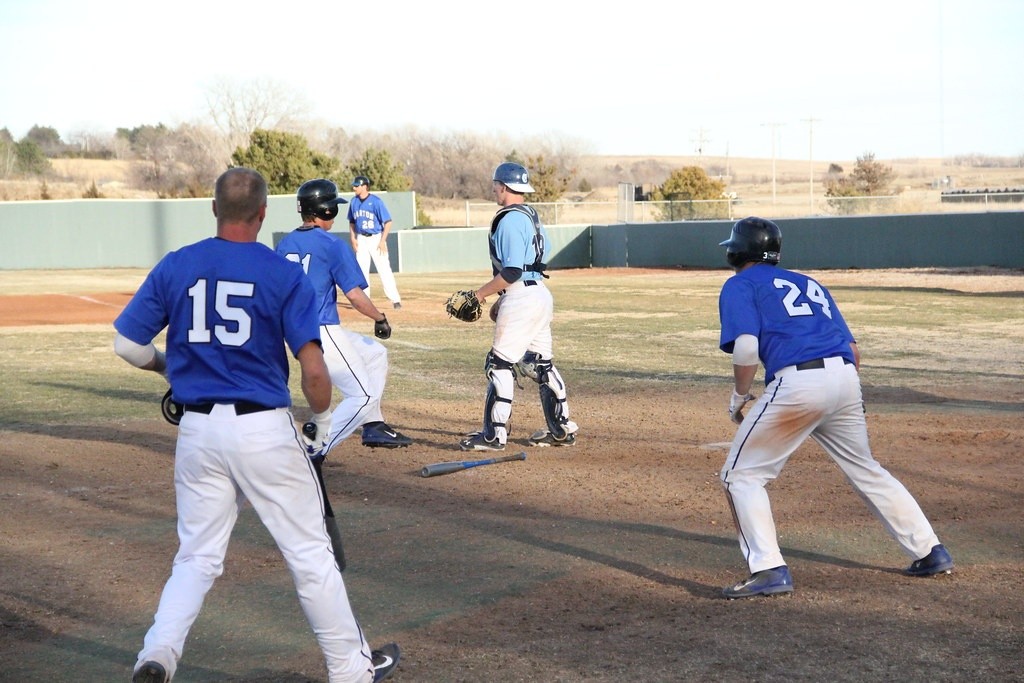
left=526, top=429, right=576, bottom=447
left=362, top=422, right=412, bottom=449
left=722, top=566, right=794, bottom=598
left=906, top=543, right=953, bottom=575
left=458, top=431, right=506, bottom=451
left=131, top=661, right=166, bottom=683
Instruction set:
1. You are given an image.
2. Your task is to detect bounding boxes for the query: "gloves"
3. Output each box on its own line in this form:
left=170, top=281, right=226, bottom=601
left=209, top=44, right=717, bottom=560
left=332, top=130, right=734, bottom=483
left=728, top=386, right=755, bottom=425
left=374, top=313, right=392, bottom=339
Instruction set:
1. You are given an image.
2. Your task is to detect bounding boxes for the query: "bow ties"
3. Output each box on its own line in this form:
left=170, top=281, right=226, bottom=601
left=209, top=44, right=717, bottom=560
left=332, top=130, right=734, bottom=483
left=371, top=643, right=401, bottom=683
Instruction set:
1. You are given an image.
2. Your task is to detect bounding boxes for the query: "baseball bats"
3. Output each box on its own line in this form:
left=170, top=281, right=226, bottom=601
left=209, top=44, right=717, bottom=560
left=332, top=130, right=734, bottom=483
left=301, top=421, right=347, bottom=573
left=421, top=452, right=527, bottom=478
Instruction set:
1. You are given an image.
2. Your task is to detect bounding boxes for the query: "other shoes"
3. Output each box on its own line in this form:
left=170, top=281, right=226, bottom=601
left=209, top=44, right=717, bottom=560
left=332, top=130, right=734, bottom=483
left=394, top=302, right=401, bottom=309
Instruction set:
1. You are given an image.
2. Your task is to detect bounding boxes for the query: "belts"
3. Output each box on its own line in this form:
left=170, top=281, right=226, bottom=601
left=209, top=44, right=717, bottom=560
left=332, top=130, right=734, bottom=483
left=498, top=279, right=537, bottom=295
left=184, top=400, right=275, bottom=416
left=772, top=356, right=853, bottom=378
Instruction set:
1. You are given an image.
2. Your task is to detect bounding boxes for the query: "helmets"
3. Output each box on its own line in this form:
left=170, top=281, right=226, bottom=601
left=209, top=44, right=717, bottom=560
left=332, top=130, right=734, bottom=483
left=718, top=216, right=782, bottom=267
left=296, top=178, right=347, bottom=221
left=352, top=176, right=369, bottom=186
left=493, top=162, right=536, bottom=192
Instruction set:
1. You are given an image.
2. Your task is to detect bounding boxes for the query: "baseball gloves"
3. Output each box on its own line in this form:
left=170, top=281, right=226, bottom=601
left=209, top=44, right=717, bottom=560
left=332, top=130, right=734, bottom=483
left=446, top=290, right=482, bottom=323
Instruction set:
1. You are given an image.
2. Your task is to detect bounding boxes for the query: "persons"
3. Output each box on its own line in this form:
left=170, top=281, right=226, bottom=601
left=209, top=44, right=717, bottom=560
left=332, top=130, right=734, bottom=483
left=112, top=168, right=399, bottom=683
left=443, top=163, right=579, bottom=453
left=273, top=179, right=413, bottom=466
left=346, top=176, right=402, bottom=310
left=717, top=216, right=954, bottom=598
